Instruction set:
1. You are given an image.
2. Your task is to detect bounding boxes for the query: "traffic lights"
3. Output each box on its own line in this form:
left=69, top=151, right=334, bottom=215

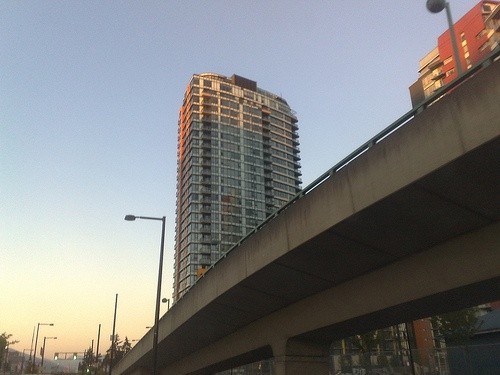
left=73, top=352, right=77, bottom=360
left=55, top=353, right=59, bottom=360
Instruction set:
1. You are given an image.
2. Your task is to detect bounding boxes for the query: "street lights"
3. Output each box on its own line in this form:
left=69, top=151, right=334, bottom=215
left=124, top=215, right=166, bottom=374
left=31, top=323, right=53, bottom=375
left=427, top=0, right=468, bottom=82
left=41, top=337, right=57, bottom=373
